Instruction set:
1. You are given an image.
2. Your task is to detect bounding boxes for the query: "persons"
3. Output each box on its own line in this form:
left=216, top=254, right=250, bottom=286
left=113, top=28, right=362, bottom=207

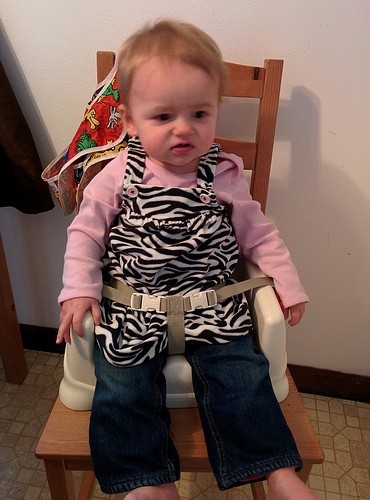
left=53, top=19, right=319, bottom=500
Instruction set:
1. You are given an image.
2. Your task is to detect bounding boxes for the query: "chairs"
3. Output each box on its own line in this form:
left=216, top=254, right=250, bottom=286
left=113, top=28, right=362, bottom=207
left=34, top=52, right=324, bottom=500
